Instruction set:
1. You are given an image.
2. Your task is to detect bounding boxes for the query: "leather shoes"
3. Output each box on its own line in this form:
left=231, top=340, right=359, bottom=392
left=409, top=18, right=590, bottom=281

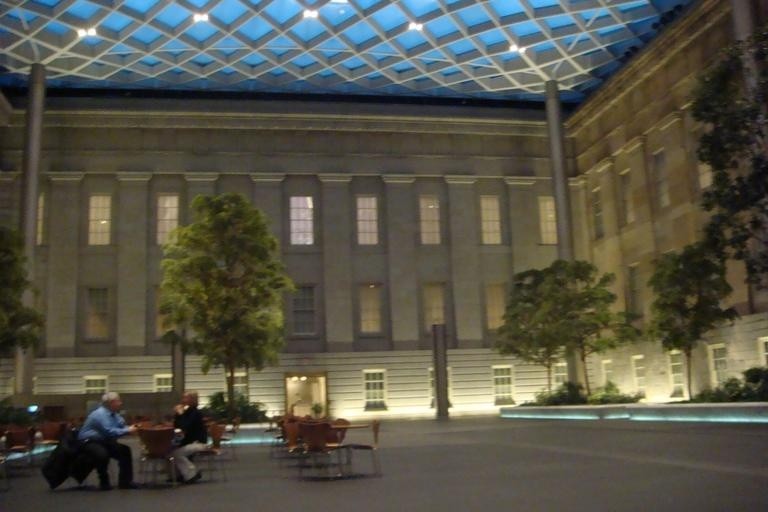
left=119, top=479, right=137, bottom=489
left=185, top=472, right=202, bottom=484
left=100, top=483, right=115, bottom=490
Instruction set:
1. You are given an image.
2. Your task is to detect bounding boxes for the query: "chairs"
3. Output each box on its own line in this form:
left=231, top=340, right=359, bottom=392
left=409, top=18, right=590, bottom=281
left=0, top=413, right=242, bottom=492
left=264, top=415, right=381, bottom=480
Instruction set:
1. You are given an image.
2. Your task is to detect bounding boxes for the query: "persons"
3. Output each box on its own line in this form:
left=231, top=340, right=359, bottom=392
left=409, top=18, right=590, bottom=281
left=77, top=391, right=144, bottom=489
left=163, top=389, right=208, bottom=484
